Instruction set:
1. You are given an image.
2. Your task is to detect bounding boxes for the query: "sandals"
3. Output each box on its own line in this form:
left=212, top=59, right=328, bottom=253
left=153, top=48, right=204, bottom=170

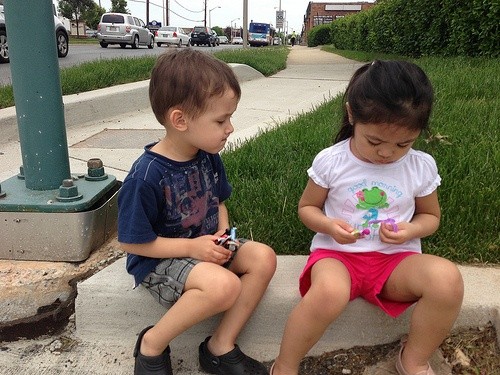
left=133, top=325, right=173, bottom=375
left=199, top=336, right=269, bottom=375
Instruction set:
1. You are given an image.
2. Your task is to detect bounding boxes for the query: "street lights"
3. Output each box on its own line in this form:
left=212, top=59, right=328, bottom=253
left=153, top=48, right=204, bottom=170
left=231, top=17, right=240, bottom=42
left=209, top=6, right=222, bottom=30
left=273, top=6, right=286, bottom=46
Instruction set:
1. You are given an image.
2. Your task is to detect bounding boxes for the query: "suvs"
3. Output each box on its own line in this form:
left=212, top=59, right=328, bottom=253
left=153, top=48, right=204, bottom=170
left=189, top=25, right=216, bottom=47
left=96, top=12, right=155, bottom=49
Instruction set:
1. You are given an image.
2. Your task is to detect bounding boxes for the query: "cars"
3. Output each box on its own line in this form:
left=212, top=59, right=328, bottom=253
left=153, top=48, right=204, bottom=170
left=0, top=0, right=70, bottom=64
left=154, top=25, right=191, bottom=48
left=86, top=29, right=96, bottom=38
left=232, top=36, right=244, bottom=45
left=219, top=35, right=229, bottom=44
left=273, top=36, right=283, bottom=46
left=211, top=29, right=220, bottom=46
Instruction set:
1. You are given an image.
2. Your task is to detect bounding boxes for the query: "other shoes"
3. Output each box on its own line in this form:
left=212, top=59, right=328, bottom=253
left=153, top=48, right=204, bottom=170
left=269, top=362, right=275, bottom=375
left=395, top=343, right=435, bottom=375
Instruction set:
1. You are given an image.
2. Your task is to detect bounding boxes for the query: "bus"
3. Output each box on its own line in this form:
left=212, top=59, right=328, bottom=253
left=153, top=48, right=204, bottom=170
left=247, top=22, right=271, bottom=47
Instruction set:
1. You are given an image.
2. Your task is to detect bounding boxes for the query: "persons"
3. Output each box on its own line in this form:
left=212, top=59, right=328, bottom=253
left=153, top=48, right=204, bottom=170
left=117, top=49, right=277, bottom=375
left=266, top=60, right=465, bottom=375
left=291, top=31, right=296, bottom=46
